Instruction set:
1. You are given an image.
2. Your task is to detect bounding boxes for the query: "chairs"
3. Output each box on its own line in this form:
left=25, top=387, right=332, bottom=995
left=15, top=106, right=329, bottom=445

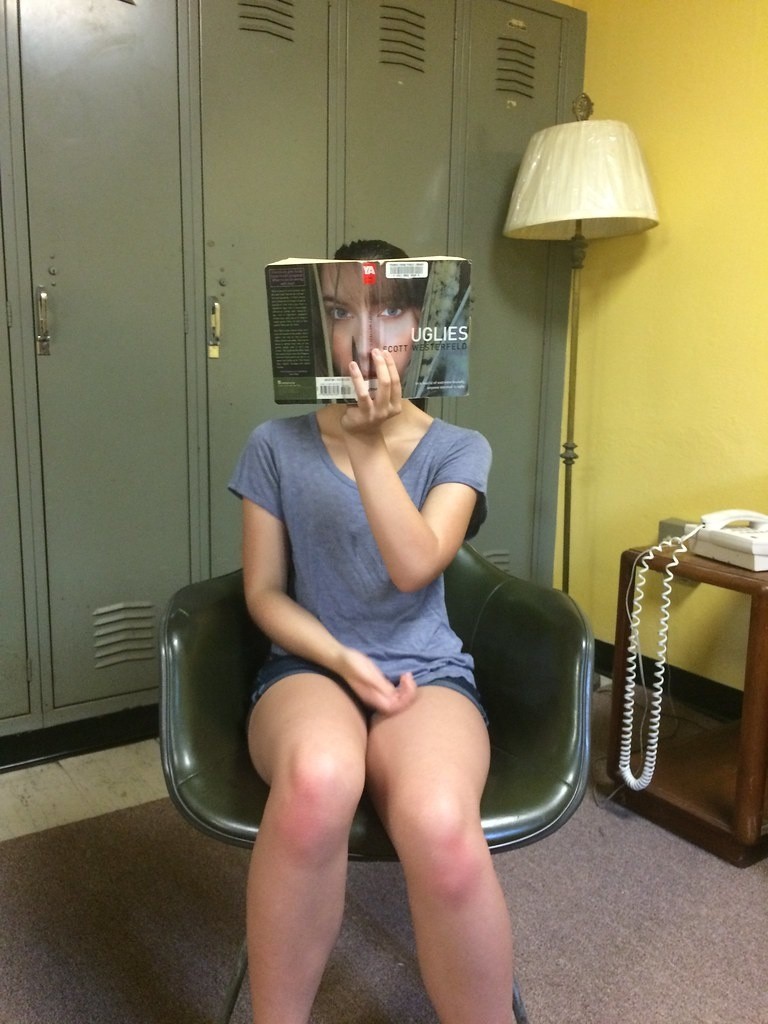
left=164, top=540, right=596, bottom=1024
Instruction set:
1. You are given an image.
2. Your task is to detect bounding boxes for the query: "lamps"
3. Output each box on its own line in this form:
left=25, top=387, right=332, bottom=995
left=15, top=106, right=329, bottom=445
left=504, top=93, right=657, bottom=590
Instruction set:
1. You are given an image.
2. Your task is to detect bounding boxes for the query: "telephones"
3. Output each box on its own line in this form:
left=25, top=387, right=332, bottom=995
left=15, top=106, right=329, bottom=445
left=684, top=509, right=768, bottom=572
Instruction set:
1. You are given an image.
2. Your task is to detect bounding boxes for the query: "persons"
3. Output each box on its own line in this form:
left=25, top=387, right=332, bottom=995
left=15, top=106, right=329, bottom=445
left=318, top=261, right=428, bottom=399
left=226, top=238, right=517, bottom=1024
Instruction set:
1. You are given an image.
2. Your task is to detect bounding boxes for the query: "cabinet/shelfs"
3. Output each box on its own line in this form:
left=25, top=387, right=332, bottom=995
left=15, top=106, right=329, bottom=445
left=0, top=0, right=590, bottom=742
left=605, top=546, right=766, bottom=868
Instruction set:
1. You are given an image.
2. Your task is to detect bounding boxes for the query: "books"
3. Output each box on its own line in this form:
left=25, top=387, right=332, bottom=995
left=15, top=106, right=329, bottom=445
left=263, top=255, right=472, bottom=405
